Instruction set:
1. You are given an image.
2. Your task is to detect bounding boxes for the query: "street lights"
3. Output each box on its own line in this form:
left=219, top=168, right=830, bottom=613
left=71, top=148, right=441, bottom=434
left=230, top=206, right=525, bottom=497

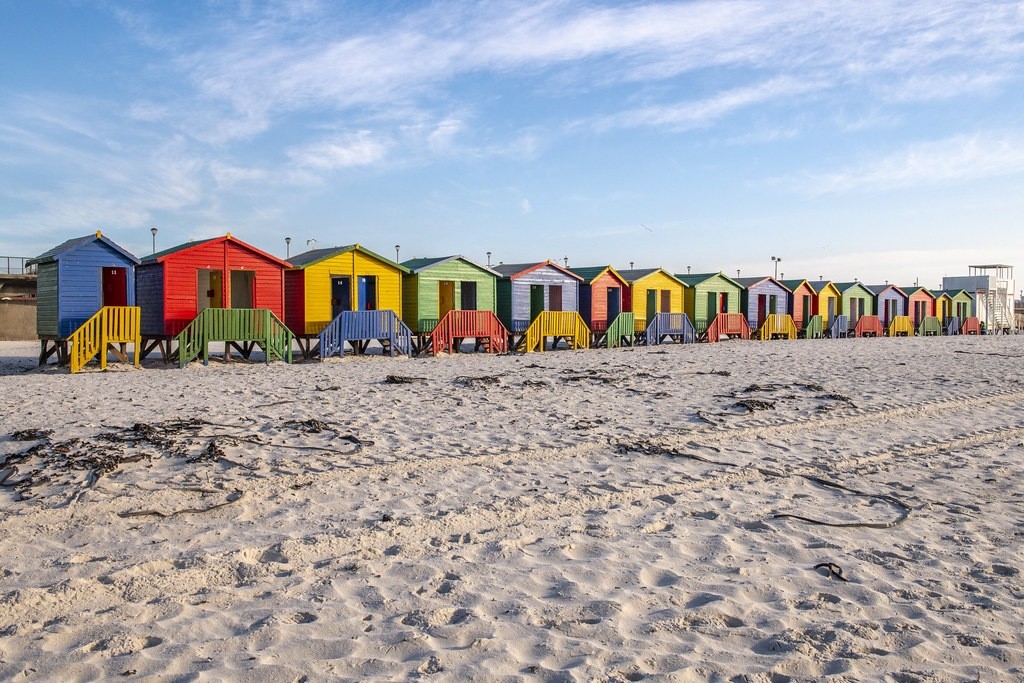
left=820, top=275, right=942, bottom=290
left=737, top=270, right=740, bottom=277
left=781, top=273, right=783, bottom=279
left=285, top=238, right=291, bottom=259
left=487, top=252, right=491, bottom=268
left=151, top=228, right=157, bottom=254
left=395, top=245, right=399, bottom=264
left=772, top=257, right=781, bottom=280
left=630, top=262, right=633, bottom=270
left=563, top=257, right=568, bottom=269
left=687, top=266, right=691, bottom=275
